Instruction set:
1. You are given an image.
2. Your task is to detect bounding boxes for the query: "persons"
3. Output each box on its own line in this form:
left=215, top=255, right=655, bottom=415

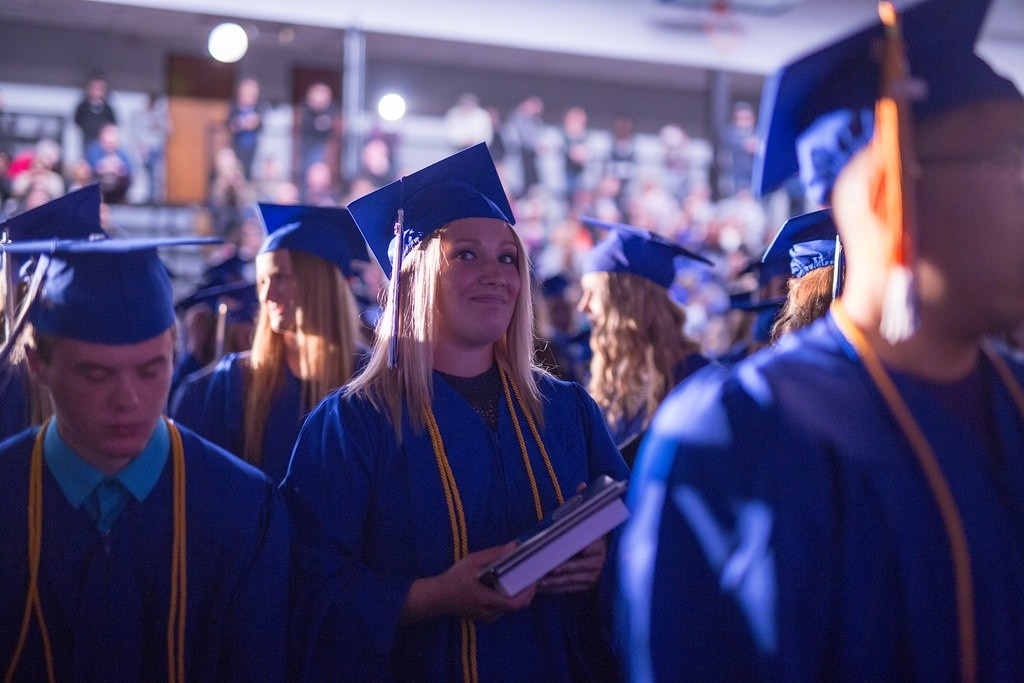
left=0, top=69, right=846, bottom=683
left=610, top=0, right=1024, bottom=673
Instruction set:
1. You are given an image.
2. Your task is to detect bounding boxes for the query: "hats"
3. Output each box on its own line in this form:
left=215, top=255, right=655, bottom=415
left=0, top=179, right=108, bottom=244
left=762, top=208, right=845, bottom=302
left=258, top=201, right=371, bottom=276
left=345, top=143, right=516, bottom=369
left=752, top=0, right=1024, bottom=344
left=578, top=214, right=715, bottom=288
left=3, top=237, right=225, bottom=347
